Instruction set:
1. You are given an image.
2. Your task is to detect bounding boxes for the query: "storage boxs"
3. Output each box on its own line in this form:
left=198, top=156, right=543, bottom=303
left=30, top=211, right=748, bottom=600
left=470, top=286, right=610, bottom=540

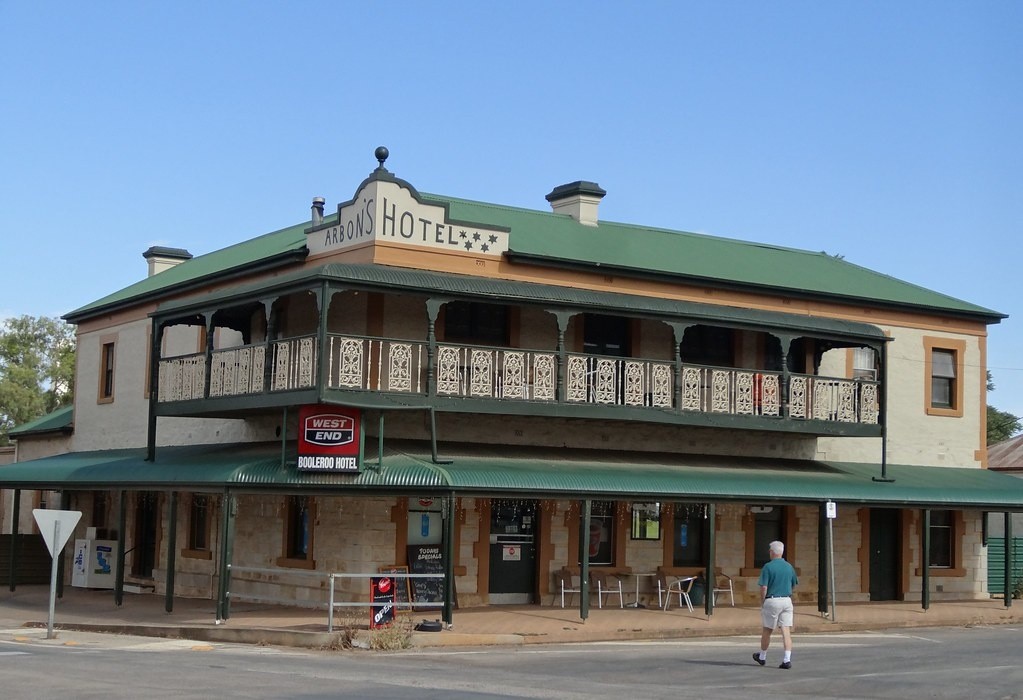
left=86, top=527, right=107, bottom=541
left=72, top=539, right=118, bottom=589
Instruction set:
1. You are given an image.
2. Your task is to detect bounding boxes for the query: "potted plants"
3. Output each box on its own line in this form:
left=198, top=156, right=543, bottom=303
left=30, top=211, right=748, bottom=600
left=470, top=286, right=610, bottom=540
left=1012, top=581, right=1023, bottom=599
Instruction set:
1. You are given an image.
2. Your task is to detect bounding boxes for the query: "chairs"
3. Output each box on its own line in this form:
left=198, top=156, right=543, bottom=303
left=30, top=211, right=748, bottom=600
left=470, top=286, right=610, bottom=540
left=590, top=570, right=623, bottom=609
left=663, top=575, right=697, bottom=613
left=551, top=570, right=580, bottom=609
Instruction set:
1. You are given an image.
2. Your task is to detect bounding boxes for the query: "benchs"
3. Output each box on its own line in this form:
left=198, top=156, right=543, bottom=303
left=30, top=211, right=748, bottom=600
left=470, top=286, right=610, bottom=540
left=657, top=567, right=735, bottom=607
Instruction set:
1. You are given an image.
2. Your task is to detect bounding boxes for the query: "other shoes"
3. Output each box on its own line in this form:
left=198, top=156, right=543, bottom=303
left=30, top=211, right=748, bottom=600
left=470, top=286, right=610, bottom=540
left=753, top=653, right=765, bottom=665
left=779, top=662, right=791, bottom=669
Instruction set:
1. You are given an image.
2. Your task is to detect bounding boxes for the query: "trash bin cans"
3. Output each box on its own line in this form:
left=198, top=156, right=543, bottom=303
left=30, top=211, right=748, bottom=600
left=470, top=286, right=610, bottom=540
left=679, top=578, right=706, bottom=609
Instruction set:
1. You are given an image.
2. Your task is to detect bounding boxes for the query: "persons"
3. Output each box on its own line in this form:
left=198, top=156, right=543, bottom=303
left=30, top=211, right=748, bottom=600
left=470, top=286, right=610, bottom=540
left=752, top=541, right=800, bottom=669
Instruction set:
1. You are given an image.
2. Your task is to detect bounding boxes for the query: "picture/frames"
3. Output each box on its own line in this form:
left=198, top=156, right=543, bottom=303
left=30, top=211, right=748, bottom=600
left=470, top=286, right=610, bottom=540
left=379, top=565, right=413, bottom=613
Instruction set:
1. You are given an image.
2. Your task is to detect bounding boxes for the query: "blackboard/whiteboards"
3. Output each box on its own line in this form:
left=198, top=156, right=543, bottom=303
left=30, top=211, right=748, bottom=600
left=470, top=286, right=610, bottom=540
left=406, top=544, right=458, bottom=612
left=378, top=566, right=413, bottom=613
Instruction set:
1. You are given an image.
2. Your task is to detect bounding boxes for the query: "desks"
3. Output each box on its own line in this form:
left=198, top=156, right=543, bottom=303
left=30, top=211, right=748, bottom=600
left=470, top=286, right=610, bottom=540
left=618, top=572, right=657, bottom=609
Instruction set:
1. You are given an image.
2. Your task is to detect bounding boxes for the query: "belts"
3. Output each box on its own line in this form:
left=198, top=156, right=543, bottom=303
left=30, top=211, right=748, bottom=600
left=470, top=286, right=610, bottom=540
left=765, top=596, right=790, bottom=599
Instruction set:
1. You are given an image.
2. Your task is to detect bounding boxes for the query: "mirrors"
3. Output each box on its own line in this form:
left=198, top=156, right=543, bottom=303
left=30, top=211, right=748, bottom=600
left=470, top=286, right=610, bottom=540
left=631, top=502, right=661, bottom=540
